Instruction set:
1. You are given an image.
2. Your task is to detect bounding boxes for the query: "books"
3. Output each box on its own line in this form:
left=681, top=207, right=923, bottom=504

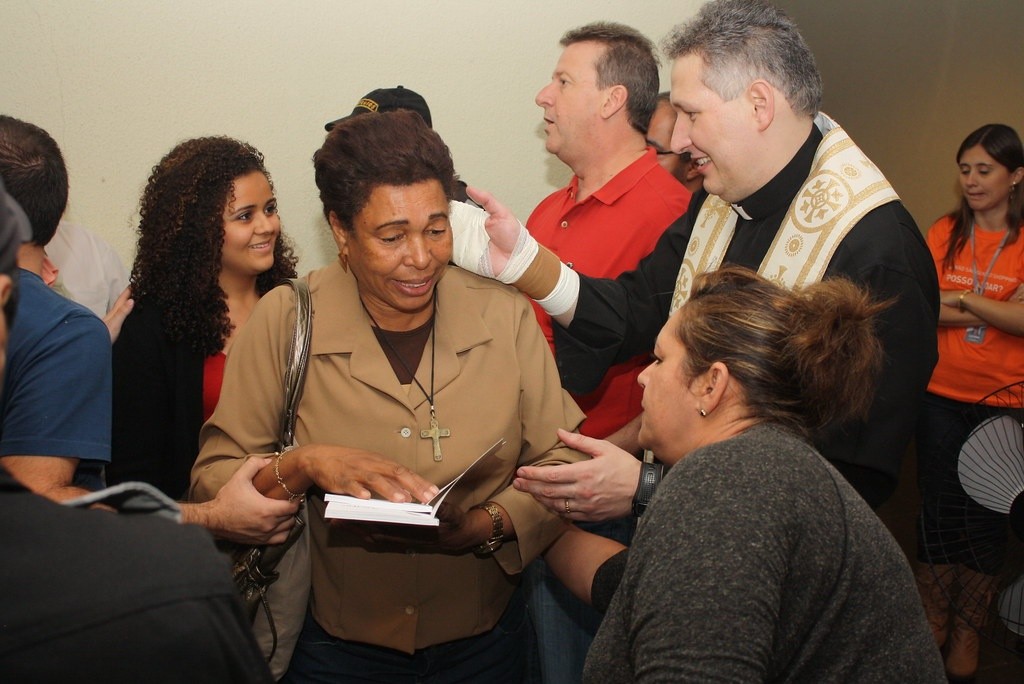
left=324, top=438, right=507, bottom=527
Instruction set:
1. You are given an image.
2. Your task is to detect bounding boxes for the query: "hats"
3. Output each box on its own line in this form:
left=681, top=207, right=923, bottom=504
left=0, top=184, right=34, bottom=273
left=325, top=85, right=432, bottom=132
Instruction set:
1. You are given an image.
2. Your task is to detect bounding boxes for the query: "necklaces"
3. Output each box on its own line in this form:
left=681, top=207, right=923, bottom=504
left=359, top=287, right=451, bottom=461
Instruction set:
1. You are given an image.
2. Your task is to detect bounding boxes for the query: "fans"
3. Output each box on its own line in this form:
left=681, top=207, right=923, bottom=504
left=920, top=381, right=1024, bottom=659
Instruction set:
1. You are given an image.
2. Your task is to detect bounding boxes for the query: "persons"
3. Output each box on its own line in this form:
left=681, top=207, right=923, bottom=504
left=190, top=113, right=591, bottom=684
left=972, top=327, right=979, bottom=336
left=645, top=93, right=704, bottom=193
left=325, top=86, right=432, bottom=131
left=917, top=124, right=1024, bottom=684
left=524, top=21, right=692, bottom=684
left=0, top=114, right=299, bottom=684
left=447, top=1, right=940, bottom=537
left=543, top=268, right=946, bottom=684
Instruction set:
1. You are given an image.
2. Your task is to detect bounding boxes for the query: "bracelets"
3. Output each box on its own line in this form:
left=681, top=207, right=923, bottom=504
left=959, top=289, right=973, bottom=312
left=274, top=449, right=306, bottom=503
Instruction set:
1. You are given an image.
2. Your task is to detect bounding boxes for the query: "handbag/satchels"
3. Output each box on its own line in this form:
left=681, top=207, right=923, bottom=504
left=219, top=277, right=315, bottom=682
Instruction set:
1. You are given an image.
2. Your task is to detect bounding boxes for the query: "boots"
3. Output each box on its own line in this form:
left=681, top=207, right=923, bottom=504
left=947, top=566, right=998, bottom=678
left=916, top=562, right=954, bottom=647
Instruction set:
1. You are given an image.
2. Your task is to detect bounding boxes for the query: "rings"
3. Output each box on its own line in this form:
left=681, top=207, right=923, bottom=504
left=565, top=499, right=570, bottom=513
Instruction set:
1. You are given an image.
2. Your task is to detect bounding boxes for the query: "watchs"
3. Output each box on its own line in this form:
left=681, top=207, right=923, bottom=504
left=633, top=461, right=665, bottom=522
left=470, top=504, right=506, bottom=555
